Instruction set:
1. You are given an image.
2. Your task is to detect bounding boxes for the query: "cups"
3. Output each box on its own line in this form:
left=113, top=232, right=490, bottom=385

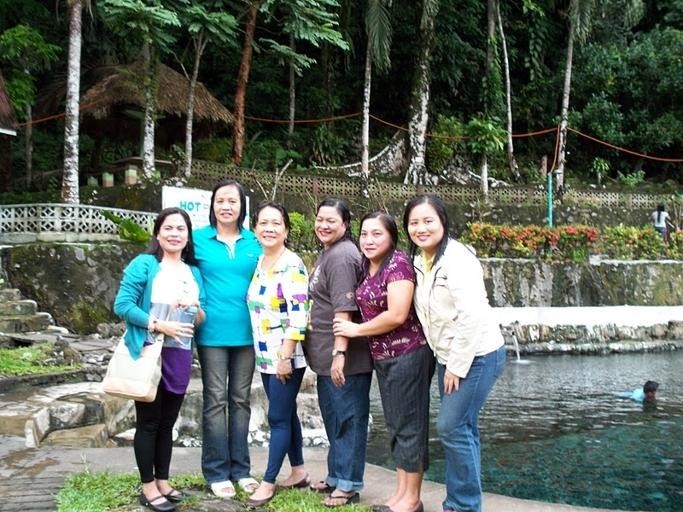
left=175, top=304, right=198, bottom=345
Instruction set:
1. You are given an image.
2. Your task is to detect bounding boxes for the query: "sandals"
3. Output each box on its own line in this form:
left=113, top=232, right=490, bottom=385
left=244, top=486, right=277, bottom=508
left=210, top=480, right=236, bottom=497
left=275, top=473, right=311, bottom=490
left=238, top=478, right=260, bottom=494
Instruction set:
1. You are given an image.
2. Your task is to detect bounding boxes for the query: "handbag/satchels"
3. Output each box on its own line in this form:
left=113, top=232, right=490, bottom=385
left=101, top=334, right=165, bottom=403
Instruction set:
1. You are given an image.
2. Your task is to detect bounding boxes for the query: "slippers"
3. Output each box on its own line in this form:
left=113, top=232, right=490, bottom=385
left=320, top=492, right=360, bottom=509
left=309, top=480, right=336, bottom=494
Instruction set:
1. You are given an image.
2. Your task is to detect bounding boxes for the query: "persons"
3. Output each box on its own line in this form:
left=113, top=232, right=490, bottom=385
left=113, top=208, right=203, bottom=511
left=631, top=379, right=658, bottom=403
left=303, top=198, right=371, bottom=508
left=186, top=180, right=267, bottom=501
left=243, top=200, right=313, bottom=508
left=402, top=193, right=511, bottom=509
left=331, top=211, right=438, bottom=511
left=651, top=202, right=671, bottom=247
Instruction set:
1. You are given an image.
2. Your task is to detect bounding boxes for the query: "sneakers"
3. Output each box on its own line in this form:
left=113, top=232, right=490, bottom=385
left=139, top=493, right=176, bottom=512
left=369, top=500, right=424, bottom=512
left=162, top=489, right=186, bottom=503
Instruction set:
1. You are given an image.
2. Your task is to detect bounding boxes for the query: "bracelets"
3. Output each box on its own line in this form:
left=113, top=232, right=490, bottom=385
left=276, top=351, right=293, bottom=359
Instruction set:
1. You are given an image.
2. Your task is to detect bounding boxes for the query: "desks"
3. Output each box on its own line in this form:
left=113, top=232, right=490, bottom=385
left=112, top=155, right=172, bottom=178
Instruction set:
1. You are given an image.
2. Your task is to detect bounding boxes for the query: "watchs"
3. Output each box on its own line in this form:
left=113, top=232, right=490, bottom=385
left=149, top=318, right=157, bottom=332
left=331, top=348, right=345, bottom=357
